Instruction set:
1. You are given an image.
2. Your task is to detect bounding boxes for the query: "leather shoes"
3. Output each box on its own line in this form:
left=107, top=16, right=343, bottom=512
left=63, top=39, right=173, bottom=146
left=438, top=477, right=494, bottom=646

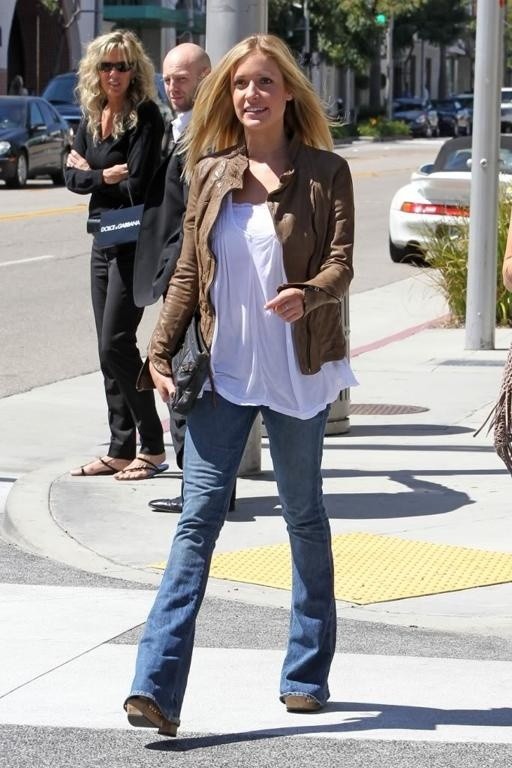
left=148, top=495, right=184, bottom=512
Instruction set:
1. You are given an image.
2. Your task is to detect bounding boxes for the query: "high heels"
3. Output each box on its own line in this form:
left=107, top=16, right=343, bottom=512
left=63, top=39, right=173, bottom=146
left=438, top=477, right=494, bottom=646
left=127, top=696, right=178, bottom=736
left=283, top=695, right=321, bottom=711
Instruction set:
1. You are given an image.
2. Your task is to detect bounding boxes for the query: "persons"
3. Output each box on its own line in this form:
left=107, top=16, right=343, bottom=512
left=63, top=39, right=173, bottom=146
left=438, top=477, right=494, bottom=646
left=131, top=42, right=235, bottom=514
left=63, top=28, right=165, bottom=480
left=123, top=34, right=355, bottom=738
left=473, top=209, right=512, bottom=477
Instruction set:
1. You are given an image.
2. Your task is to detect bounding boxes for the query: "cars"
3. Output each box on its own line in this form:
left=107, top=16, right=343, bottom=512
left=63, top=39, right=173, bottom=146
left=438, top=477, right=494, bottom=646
left=0, top=71, right=173, bottom=188
left=389, top=87, right=512, bottom=264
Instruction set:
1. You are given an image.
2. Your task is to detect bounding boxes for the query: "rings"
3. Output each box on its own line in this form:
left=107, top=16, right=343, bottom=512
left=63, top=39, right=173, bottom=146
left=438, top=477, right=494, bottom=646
left=70, top=163, right=75, bottom=167
left=284, top=304, right=289, bottom=311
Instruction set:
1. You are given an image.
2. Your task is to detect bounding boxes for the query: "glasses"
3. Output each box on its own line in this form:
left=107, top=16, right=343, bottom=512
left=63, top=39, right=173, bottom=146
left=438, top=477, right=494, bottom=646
left=95, top=60, right=136, bottom=72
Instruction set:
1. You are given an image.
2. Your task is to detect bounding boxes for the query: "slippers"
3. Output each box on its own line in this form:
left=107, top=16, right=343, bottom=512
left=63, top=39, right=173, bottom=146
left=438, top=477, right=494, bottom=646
left=70, top=457, right=120, bottom=476
left=116, top=456, right=170, bottom=480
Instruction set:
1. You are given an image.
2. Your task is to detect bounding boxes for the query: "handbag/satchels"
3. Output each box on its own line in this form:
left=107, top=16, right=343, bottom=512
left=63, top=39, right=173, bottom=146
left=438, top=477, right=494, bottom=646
left=99, top=202, right=144, bottom=249
left=165, top=312, right=210, bottom=417
